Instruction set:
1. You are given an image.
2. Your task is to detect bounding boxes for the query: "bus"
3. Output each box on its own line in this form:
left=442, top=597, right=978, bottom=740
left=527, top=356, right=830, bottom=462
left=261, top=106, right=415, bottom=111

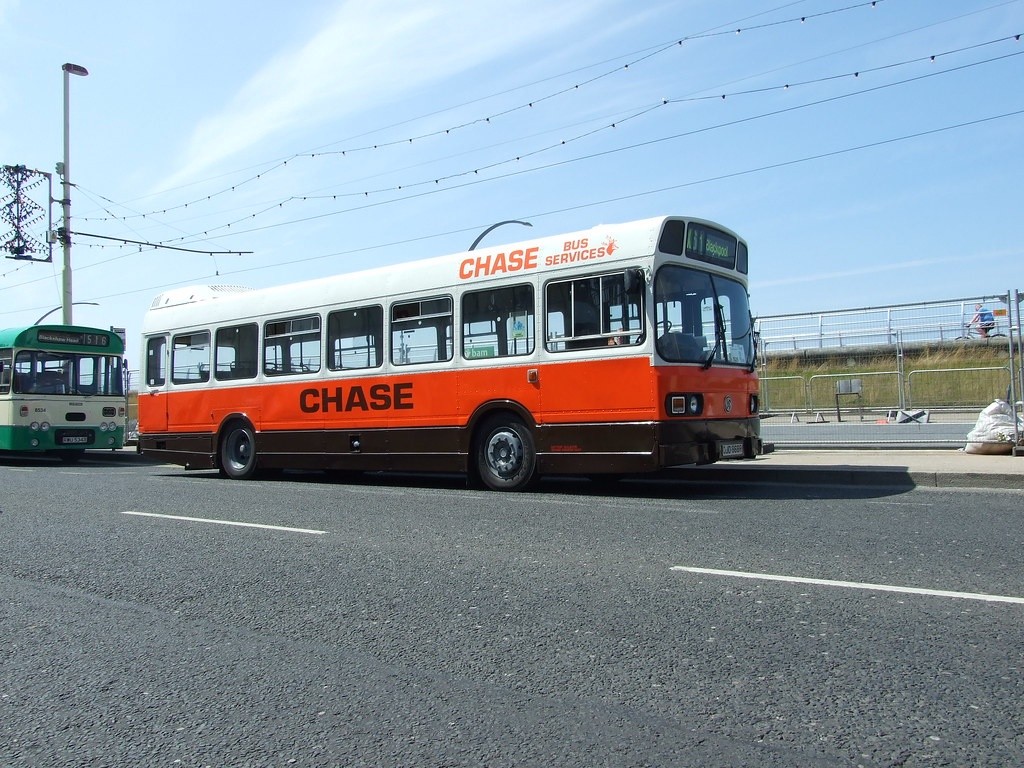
left=135, top=215, right=775, bottom=491
left=0, top=324, right=130, bottom=466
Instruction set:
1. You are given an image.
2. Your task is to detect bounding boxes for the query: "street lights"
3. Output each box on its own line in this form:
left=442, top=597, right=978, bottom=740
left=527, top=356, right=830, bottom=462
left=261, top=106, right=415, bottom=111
left=34, top=302, right=99, bottom=326
left=61, top=61, right=88, bottom=324
left=470, top=220, right=534, bottom=252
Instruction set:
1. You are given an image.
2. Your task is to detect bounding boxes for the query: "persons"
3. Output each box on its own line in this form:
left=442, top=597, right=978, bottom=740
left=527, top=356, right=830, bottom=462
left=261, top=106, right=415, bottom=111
left=969, top=304, right=995, bottom=338
left=615, top=327, right=625, bottom=344
left=608, top=338, right=617, bottom=346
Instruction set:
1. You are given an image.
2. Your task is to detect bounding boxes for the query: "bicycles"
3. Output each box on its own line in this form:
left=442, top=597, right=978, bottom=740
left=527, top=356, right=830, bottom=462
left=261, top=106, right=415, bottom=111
left=955, top=321, right=1007, bottom=340
